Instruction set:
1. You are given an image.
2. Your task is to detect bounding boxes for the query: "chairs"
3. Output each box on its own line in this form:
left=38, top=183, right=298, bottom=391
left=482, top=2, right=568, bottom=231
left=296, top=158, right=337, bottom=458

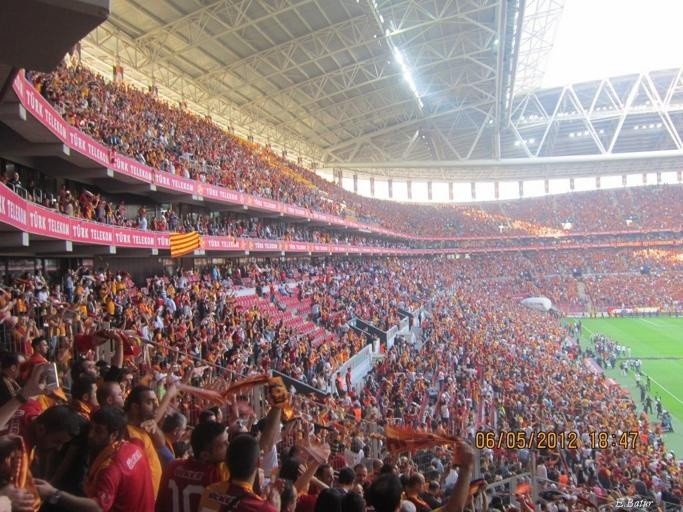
left=221, top=272, right=498, bottom=450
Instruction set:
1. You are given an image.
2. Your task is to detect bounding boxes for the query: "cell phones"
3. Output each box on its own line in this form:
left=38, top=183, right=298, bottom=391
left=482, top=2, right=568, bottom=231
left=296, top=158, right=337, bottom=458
left=42, top=362, right=59, bottom=388
